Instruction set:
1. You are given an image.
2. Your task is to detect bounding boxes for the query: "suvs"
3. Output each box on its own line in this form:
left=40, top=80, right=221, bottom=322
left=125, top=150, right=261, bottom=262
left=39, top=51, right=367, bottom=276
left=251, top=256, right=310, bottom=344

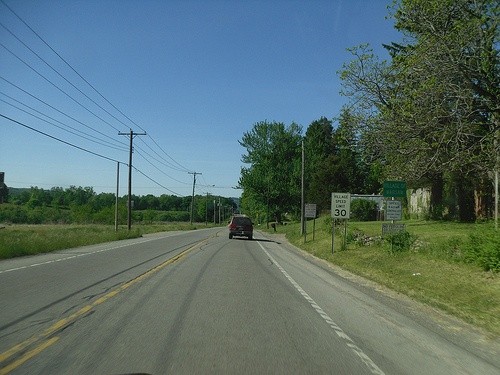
left=228, top=216, right=254, bottom=240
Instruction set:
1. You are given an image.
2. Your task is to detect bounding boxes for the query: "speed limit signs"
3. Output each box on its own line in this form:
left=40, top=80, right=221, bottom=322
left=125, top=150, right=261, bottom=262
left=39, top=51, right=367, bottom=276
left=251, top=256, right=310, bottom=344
left=331, top=192, right=350, bottom=219
